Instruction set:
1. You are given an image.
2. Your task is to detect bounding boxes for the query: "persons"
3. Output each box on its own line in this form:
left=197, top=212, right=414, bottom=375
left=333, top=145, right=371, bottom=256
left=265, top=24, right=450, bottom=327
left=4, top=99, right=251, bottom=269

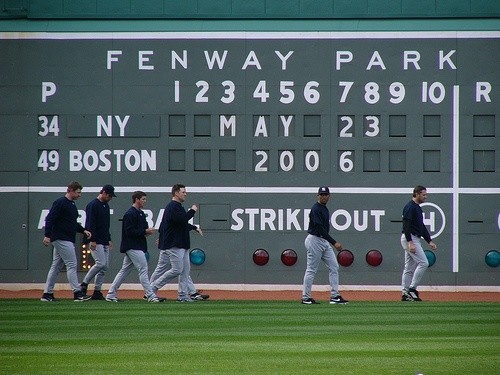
left=300, top=187, right=350, bottom=305
left=40, top=181, right=93, bottom=303
left=104, top=191, right=164, bottom=303
left=79, top=185, right=118, bottom=300
left=400, top=186, right=438, bottom=302
left=143, top=184, right=210, bottom=302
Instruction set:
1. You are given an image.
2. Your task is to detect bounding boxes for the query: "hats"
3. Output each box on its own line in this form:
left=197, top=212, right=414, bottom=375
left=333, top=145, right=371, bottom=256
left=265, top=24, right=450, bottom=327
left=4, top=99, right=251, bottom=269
left=103, top=184, right=118, bottom=197
left=318, top=186, right=330, bottom=195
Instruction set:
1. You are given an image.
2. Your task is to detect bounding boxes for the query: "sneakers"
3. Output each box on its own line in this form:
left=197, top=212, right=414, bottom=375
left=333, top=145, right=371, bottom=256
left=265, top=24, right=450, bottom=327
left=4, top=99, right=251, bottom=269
left=329, top=295, right=350, bottom=304
left=142, top=295, right=151, bottom=301
left=401, top=293, right=415, bottom=301
left=106, top=296, right=118, bottom=302
left=73, top=293, right=91, bottom=302
left=176, top=296, right=198, bottom=302
left=408, top=288, right=423, bottom=301
left=92, top=290, right=106, bottom=300
left=191, top=293, right=210, bottom=300
left=40, top=293, right=56, bottom=301
left=302, top=297, right=320, bottom=304
left=148, top=297, right=166, bottom=302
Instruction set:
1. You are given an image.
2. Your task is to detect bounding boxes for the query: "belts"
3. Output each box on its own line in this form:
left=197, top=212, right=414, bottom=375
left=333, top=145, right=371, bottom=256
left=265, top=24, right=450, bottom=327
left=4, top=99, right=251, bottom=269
left=412, top=233, right=421, bottom=238
